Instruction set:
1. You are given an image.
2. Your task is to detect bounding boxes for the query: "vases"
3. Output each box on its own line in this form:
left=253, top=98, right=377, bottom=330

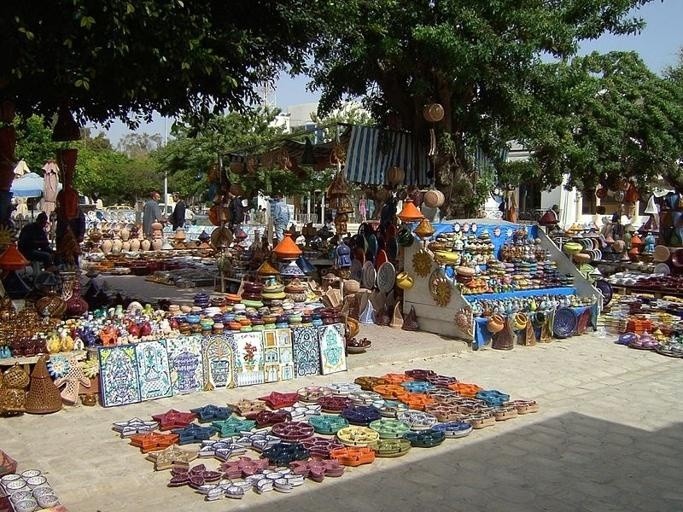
left=64, top=281, right=88, bottom=319
left=89, top=222, right=163, bottom=255
left=487, top=311, right=547, bottom=333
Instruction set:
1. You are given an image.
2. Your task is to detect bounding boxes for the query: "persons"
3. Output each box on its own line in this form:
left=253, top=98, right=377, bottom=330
left=91, top=195, right=104, bottom=221
left=170, top=191, right=185, bottom=232
left=538, top=205, right=560, bottom=226
left=358, top=193, right=368, bottom=224
left=66, top=190, right=83, bottom=268
left=270, top=195, right=290, bottom=242
left=17, top=211, right=54, bottom=272
left=260, top=208, right=267, bottom=224
left=226, top=195, right=242, bottom=233
left=141, top=190, right=162, bottom=239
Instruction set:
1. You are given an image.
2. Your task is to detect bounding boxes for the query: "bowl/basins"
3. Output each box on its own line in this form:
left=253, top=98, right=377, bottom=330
left=0, top=468, right=62, bottom=511
left=167, top=296, right=319, bottom=337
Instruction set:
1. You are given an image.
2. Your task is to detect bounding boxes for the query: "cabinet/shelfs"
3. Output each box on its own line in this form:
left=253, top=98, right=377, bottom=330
left=403, top=218, right=605, bottom=343
